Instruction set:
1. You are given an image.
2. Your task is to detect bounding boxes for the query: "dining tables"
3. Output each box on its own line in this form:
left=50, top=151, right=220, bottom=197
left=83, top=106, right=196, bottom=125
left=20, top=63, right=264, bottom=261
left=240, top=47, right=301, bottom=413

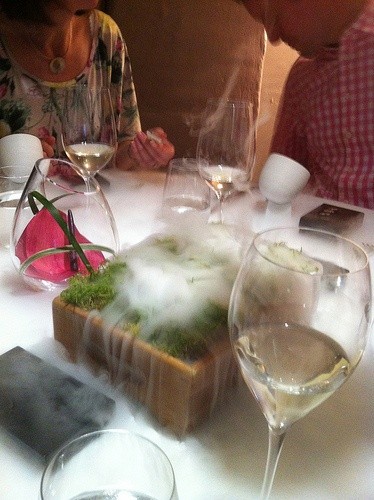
left=0, top=166, right=374, bottom=500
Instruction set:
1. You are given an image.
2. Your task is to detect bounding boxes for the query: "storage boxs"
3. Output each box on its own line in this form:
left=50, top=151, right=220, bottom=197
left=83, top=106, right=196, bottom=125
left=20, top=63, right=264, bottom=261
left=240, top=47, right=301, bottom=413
left=299, top=203, right=364, bottom=242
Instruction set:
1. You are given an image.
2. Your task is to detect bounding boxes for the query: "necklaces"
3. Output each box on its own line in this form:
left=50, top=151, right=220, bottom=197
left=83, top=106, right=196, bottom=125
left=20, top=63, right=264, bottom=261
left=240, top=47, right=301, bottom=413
left=27, top=21, right=71, bottom=75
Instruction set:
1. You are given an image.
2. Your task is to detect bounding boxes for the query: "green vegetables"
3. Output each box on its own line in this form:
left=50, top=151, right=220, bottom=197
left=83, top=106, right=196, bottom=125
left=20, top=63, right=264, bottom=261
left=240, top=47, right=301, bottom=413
left=59, top=221, right=315, bottom=366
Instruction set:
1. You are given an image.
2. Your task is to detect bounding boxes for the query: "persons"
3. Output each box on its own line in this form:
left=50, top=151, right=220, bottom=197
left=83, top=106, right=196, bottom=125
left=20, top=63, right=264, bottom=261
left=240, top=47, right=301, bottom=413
left=237, top=0, right=374, bottom=210
left=0, top=0, right=174, bottom=175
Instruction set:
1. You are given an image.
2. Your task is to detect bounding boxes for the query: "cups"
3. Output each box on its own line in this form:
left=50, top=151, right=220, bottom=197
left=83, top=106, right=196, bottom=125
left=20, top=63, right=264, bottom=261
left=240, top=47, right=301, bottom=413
left=0, top=166, right=47, bottom=249
left=259, top=152, right=311, bottom=205
left=1, top=134, right=44, bottom=184
left=161, top=157, right=211, bottom=224
left=40, top=428, right=178, bottom=500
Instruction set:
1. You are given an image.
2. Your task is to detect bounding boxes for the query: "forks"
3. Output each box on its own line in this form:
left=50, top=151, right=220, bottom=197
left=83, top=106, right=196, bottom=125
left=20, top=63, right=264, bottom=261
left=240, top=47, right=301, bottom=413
left=354, top=241, right=374, bottom=257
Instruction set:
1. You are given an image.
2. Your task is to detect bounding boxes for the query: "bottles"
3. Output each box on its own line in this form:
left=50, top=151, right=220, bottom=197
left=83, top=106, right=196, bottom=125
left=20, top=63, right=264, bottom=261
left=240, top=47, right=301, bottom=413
left=8, top=157, right=120, bottom=293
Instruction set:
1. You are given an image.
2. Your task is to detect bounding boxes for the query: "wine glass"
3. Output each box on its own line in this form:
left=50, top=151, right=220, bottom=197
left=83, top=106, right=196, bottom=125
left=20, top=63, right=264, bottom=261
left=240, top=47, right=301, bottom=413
left=227, top=225, right=371, bottom=500
left=61, top=84, right=118, bottom=214
left=195, top=98, right=257, bottom=224
